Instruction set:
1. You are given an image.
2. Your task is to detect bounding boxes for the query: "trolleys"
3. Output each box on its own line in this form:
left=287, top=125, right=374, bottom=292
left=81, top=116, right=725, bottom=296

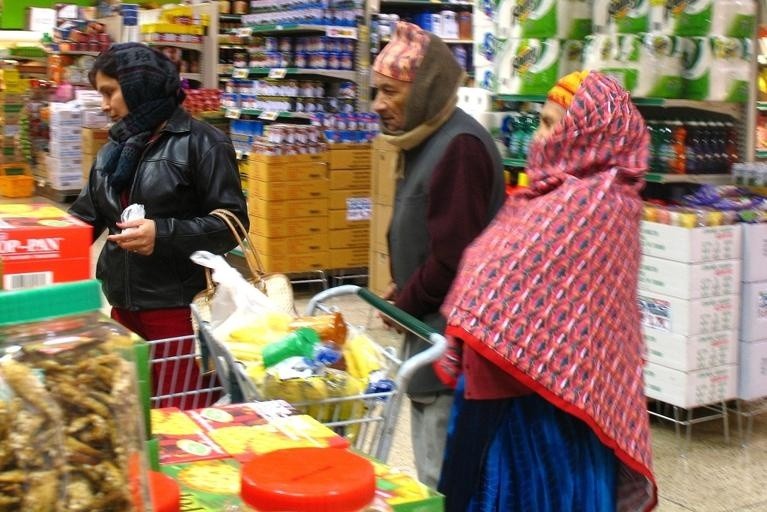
left=146, top=284, right=448, bottom=465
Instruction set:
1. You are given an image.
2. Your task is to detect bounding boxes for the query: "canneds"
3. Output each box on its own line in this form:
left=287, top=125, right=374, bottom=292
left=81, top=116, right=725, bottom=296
left=220, top=0, right=383, bottom=156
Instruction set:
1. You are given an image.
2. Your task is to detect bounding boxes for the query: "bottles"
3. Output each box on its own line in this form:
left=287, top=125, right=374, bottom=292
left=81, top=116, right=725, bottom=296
left=0, top=279, right=150, bottom=512
left=643, top=121, right=732, bottom=171
left=221, top=445, right=396, bottom=512
left=509, top=110, right=539, bottom=157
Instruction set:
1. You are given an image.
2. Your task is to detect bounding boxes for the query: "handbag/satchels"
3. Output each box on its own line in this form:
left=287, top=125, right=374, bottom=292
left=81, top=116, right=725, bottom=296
left=187, top=206, right=299, bottom=377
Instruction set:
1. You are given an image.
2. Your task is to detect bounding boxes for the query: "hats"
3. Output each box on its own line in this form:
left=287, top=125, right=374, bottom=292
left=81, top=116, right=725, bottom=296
left=371, top=21, right=430, bottom=85
left=546, top=67, right=588, bottom=113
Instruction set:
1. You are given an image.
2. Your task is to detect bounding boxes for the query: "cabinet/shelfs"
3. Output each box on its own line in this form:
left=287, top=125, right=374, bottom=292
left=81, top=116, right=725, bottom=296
left=144, top=3, right=472, bottom=122
left=495, top=95, right=740, bottom=186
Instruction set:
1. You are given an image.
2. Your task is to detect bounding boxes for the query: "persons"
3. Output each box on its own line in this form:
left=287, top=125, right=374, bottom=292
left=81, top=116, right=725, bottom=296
left=65, top=42, right=252, bottom=416
left=433, top=65, right=658, bottom=510
left=370, top=20, right=505, bottom=493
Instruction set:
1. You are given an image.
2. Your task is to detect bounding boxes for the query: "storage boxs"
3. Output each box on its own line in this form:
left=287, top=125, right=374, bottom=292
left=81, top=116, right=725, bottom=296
left=50, top=89, right=110, bottom=192
left=640, top=221, right=767, bottom=410
left=245, top=143, right=370, bottom=274
